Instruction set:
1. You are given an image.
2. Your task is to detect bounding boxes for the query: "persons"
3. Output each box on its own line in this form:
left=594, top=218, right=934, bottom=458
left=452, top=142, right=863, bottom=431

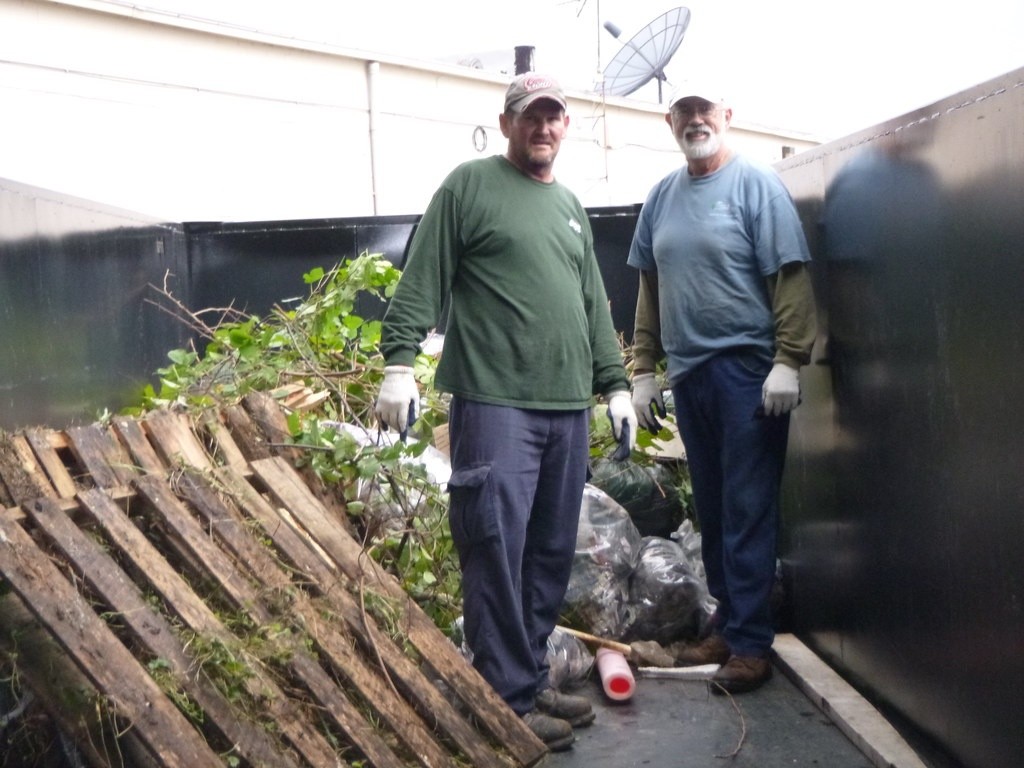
left=626, top=72, right=818, bottom=692
left=820, top=103, right=959, bottom=540
left=374, top=71, right=639, bottom=749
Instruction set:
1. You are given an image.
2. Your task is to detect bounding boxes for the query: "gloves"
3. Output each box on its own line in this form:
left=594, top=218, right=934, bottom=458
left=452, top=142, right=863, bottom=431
left=603, top=389, right=641, bottom=462
left=759, top=364, right=803, bottom=416
left=373, top=364, right=420, bottom=442
left=631, top=373, right=667, bottom=436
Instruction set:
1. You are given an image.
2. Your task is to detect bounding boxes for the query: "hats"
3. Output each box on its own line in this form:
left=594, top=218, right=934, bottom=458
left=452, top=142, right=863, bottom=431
left=503, top=71, right=567, bottom=112
left=666, top=75, right=729, bottom=110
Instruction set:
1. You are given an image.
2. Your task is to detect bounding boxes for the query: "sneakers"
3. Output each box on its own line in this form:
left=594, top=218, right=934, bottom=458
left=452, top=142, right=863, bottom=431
left=710, top=653, right=774, bottom=696
left=520, top=708, right=576, bottom=749
left=534, top=686, right=596, bottom=726
left=674, top=633, right=730, bottom=667
